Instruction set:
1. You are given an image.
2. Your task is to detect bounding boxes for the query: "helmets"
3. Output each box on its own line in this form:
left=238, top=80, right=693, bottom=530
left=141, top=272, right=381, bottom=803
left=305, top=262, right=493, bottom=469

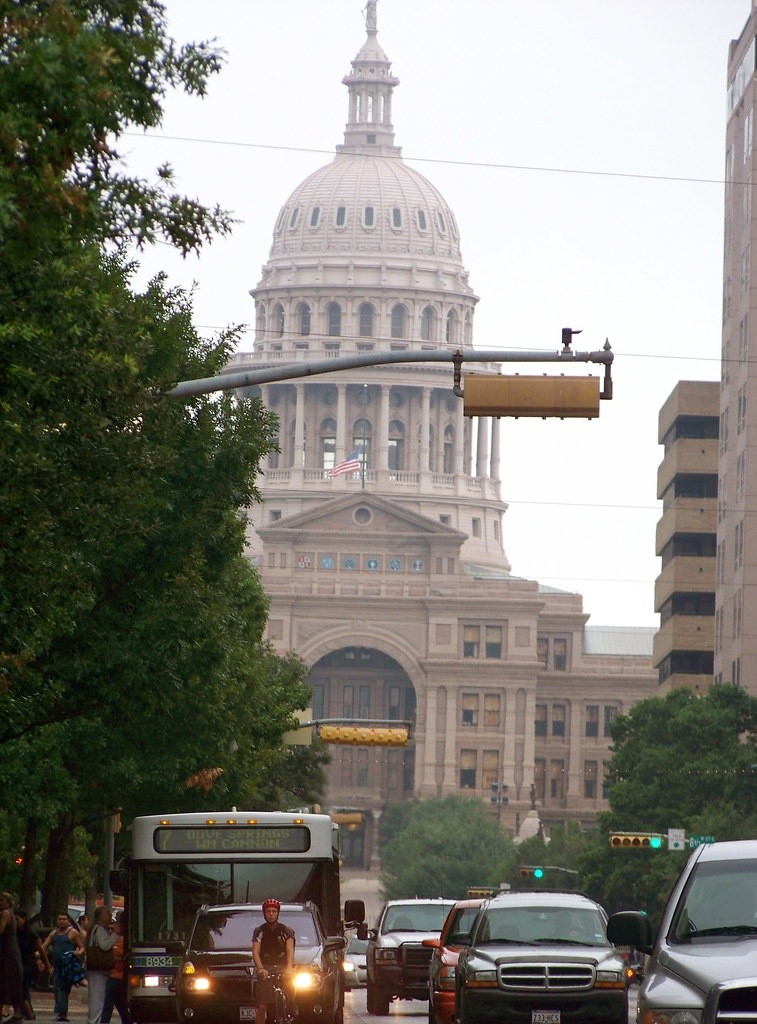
left=262, top=899, right=280, bottom=913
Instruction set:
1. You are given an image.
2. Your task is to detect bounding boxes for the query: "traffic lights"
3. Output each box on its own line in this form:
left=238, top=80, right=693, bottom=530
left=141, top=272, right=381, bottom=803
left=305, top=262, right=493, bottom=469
left=610, top=834, right=663, bottom=849
left=520, top=868, right=544, bottom=879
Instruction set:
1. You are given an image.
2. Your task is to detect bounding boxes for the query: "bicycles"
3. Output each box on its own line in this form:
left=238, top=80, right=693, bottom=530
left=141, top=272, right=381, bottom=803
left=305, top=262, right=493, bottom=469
left=255, top=970, right=299, bottom=1024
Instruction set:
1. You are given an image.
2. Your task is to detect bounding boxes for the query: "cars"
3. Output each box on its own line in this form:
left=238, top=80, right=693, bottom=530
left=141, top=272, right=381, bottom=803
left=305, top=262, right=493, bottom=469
left=22, top=904, right=124, bottom=993
left=164, top=899, right=346, bottom=1024
left=342, top=928, right=367, bottom=991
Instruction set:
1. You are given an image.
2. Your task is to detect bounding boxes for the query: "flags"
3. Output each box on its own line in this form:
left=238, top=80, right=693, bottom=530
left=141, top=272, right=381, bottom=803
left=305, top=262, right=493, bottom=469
left=328, top=446, right=360, bottom=477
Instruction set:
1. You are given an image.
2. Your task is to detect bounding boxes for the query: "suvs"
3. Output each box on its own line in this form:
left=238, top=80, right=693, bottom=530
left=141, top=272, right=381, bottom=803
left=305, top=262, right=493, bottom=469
left=422, top=897, right=496, bottom=1024
left=454, top=889, right=631, bottom=1024
left=357, top=897, right=466, bottom=1017
left=604, top=839, right=757, bottom=1024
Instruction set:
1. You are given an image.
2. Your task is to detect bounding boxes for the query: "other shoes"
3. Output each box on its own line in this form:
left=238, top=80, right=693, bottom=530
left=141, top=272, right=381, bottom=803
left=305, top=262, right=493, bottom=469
left=3, top=1017, right=24, bottom=1024
left=2, top=1014, right=10, bottom=1022
left=57, top=1012, right=67, bottom=1020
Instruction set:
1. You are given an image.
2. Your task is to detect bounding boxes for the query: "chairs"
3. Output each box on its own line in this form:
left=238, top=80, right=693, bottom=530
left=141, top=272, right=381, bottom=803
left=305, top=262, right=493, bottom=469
left=494, top=924, right=519, bottom=941
left=392, top=916, right=413, bottom=929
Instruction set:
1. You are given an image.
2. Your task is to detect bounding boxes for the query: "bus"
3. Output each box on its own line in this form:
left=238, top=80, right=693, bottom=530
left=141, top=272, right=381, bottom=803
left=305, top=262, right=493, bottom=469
left=107, top=803, right=366, bottom=1024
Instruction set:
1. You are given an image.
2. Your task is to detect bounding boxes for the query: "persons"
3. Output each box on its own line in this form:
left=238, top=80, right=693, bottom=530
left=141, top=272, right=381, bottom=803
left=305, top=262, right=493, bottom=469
left=87, top=907, right=124, bottom=1024
left=76, top=915, right=88, bottom=987
left=35, top=912, right=85, bottom=1021
left=2, top=909, right=52, bottom=1020
left=555, top=912, right=584, bottom=938
left=529, top=783, right=537, bottom=810
left=251, top=899, right=298, bottom=1024
left=0, top=892, right=24, bottom=1024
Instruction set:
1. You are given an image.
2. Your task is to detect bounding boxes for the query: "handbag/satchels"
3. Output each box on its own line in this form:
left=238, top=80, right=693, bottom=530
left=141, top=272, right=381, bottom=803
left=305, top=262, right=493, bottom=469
left=57, top=951, right=86, bottom=988
left=85, top=926, right=115, bottom=970
